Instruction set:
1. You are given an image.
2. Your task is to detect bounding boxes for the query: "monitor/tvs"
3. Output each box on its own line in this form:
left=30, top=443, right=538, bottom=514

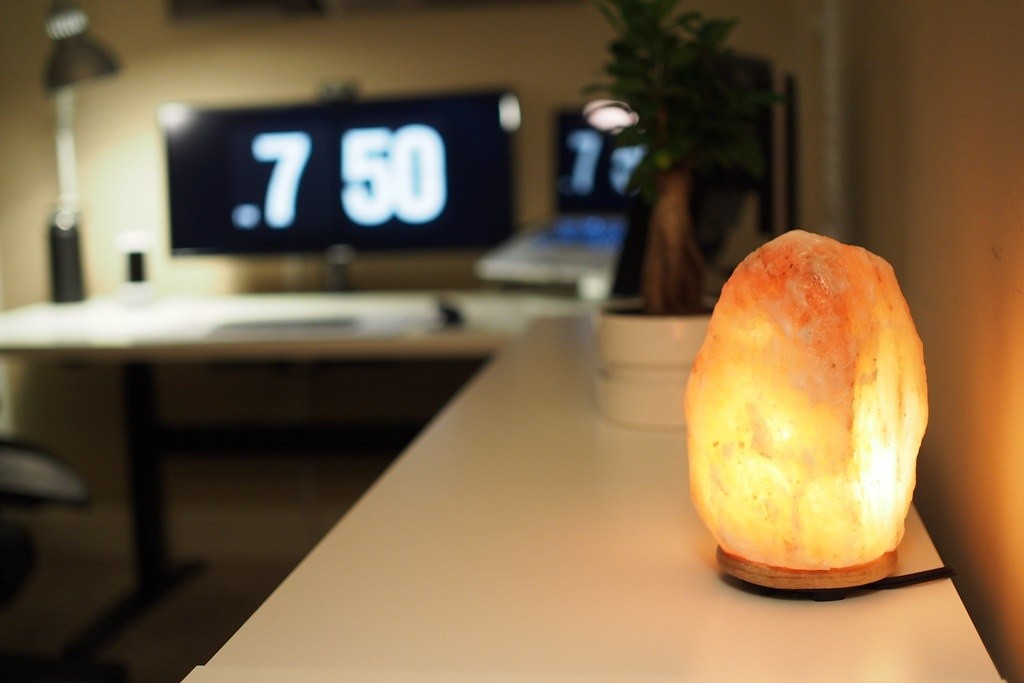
left=165, top=90, right=520, bottom=251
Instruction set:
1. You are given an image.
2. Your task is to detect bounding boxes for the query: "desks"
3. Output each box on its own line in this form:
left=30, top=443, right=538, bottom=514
left=181, top=313, right=1001, bottom=683
left=0, top=296, right=598, bottom=659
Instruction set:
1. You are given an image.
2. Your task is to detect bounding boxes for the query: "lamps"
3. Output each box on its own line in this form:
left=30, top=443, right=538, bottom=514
left=682, top=228, right=931, bottom=600
left=41, top=1, right=116, bottom=303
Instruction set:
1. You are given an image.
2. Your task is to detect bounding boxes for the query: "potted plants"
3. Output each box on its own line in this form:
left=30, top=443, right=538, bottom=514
left=580, top=1, right=784, bottom=312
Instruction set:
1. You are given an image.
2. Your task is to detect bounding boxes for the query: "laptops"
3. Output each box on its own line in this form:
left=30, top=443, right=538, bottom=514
left=485, top=110, right=651, bottom=284
